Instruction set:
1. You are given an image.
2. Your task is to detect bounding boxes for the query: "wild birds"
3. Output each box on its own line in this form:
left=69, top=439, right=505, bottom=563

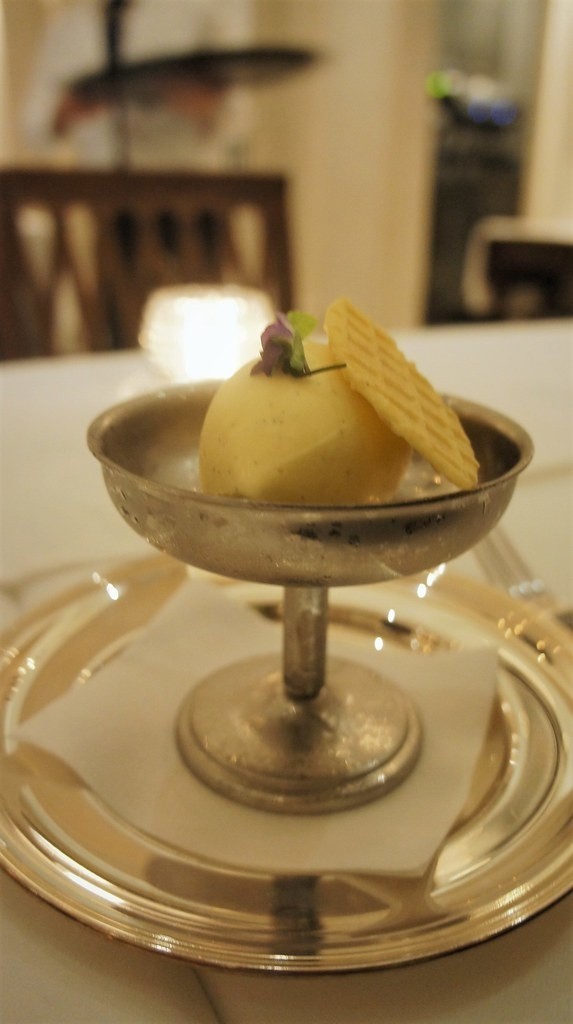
left=425, top=70, right=532, bottom=132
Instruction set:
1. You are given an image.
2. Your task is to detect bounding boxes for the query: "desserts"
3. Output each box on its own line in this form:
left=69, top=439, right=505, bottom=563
left=195, top=341, right=413, bottom=506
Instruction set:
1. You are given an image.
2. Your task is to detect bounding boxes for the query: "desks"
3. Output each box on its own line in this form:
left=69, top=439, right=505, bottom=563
left=1, top=315, right=573, bottom=1024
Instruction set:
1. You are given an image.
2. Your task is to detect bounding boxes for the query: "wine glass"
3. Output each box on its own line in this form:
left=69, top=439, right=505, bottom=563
left=82, top=381, right=539, bottom=815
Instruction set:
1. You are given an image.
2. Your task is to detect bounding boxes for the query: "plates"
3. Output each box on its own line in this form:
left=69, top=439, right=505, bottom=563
left=0, top=562, right=573, bottom=974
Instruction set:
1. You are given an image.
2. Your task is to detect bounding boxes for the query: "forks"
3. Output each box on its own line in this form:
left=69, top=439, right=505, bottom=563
left=477, top=526, right=560, bottom=619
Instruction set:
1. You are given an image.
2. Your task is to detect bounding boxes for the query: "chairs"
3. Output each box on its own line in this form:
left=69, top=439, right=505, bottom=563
left=0, top=167, right=296, bottom=364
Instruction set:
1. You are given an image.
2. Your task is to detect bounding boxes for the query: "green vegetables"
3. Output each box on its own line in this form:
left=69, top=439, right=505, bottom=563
left=278, top=309, right=347, bottom=378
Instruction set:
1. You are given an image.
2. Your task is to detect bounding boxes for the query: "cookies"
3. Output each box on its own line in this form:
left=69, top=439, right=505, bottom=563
left=325, top=296, right=480, bottom=491
